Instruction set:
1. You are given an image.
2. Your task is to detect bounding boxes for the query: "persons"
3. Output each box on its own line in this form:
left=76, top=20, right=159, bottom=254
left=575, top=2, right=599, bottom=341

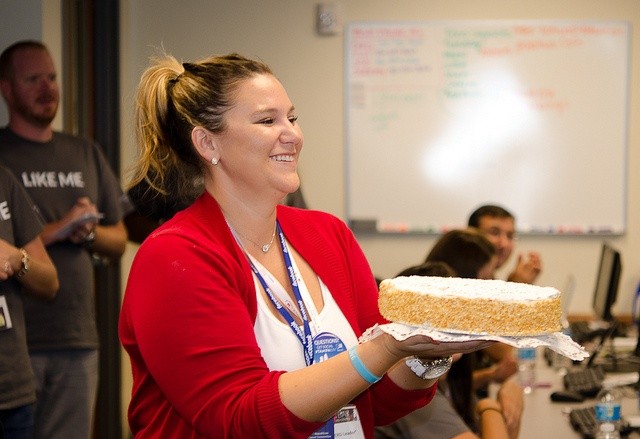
left=375, top=262, right=525, bottom=439
left=423, top=228, right=521, bottom=436
left=461, top=205, right=541, bottom=285
left=0, top=165, right=60, bottom=439
left=117, top=48, right=502, bottom=439
left=1, top=39, right=128, bottom=439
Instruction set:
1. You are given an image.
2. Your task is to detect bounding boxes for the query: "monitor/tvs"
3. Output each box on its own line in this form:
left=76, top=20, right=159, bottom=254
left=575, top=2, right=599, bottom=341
left=591, top=243, right=621, bottom=323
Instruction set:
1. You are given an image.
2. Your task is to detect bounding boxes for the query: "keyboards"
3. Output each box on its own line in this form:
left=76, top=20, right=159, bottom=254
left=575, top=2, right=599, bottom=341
left=564, top=368, right=600, bottom=396
left=569, top=405, right=632, bottom=438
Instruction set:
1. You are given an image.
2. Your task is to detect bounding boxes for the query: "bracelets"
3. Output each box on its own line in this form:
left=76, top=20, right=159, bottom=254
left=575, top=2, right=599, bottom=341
left=348, top=346, right=382, bottom=383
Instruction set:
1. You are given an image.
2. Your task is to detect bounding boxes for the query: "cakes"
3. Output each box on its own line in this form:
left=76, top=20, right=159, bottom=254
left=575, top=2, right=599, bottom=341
left=377, top=275, right=570, bottom=337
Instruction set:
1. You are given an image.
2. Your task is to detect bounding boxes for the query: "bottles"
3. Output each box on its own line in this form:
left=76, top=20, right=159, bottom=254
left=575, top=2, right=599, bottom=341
left=596, top=381, right=621, bottom=439
left=516, top=342, right=537, bottom=394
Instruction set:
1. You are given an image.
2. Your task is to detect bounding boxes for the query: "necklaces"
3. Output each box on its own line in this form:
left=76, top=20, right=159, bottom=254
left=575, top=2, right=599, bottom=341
left=236, top=220, right=278, bottom=254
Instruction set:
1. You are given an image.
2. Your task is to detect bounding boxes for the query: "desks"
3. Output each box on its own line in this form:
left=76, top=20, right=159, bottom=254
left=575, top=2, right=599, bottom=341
left=483, top=324, right=639, bottom=439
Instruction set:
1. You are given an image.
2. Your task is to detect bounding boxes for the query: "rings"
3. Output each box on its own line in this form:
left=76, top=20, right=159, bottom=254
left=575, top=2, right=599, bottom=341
left=4, top=262, right=9, bottom=272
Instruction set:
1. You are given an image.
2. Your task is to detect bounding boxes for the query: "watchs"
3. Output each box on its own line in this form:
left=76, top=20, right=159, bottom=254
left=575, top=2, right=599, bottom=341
left=15, top=248, right=31, bottom=280
left=404, top=354, right=453, bottom=380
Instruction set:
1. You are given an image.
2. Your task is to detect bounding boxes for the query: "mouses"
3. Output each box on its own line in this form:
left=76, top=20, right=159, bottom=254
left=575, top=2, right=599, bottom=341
left=551, top=391, right=584, bottom=402
